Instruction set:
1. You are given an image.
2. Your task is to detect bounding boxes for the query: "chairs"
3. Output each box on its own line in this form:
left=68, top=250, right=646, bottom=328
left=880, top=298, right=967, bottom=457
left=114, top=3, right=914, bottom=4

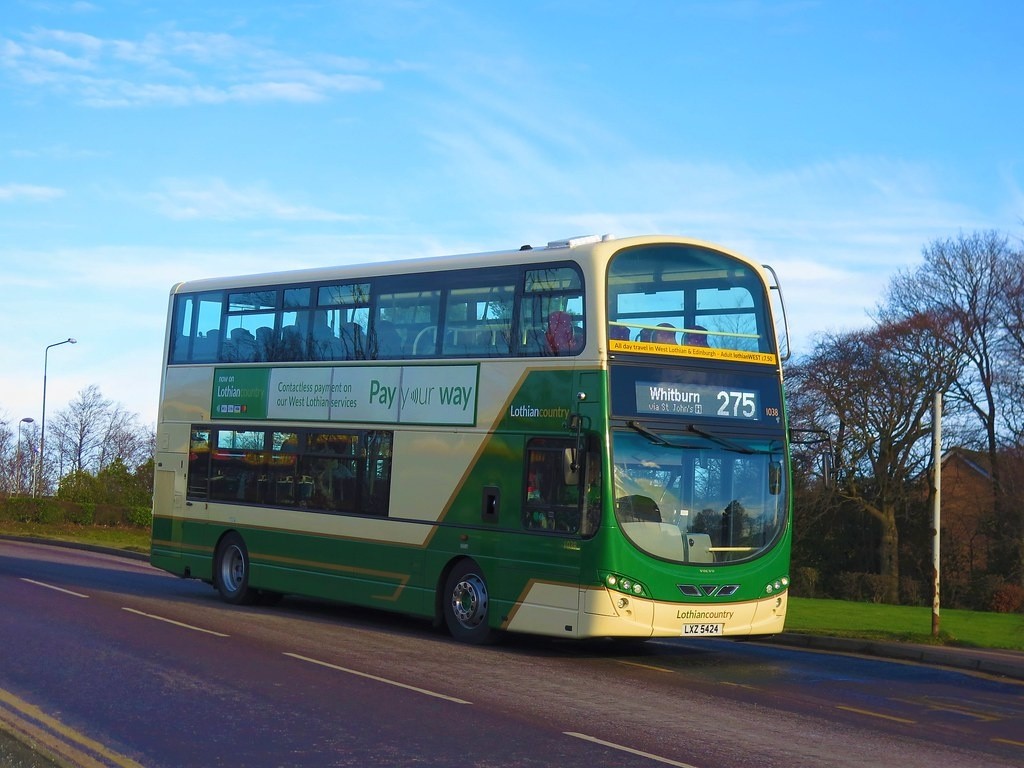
left=175, top=311, right=710, bottom=361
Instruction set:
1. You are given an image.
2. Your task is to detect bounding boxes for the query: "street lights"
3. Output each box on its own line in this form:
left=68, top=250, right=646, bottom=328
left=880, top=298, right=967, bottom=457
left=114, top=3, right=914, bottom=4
left=38, top=338, right=78, bottom=499
left=16, top=418, right=34, bottom=499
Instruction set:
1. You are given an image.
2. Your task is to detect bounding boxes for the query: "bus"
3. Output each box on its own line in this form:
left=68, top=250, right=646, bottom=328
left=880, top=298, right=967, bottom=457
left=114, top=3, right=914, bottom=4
left=149, top=232, right=838, bottom=657
